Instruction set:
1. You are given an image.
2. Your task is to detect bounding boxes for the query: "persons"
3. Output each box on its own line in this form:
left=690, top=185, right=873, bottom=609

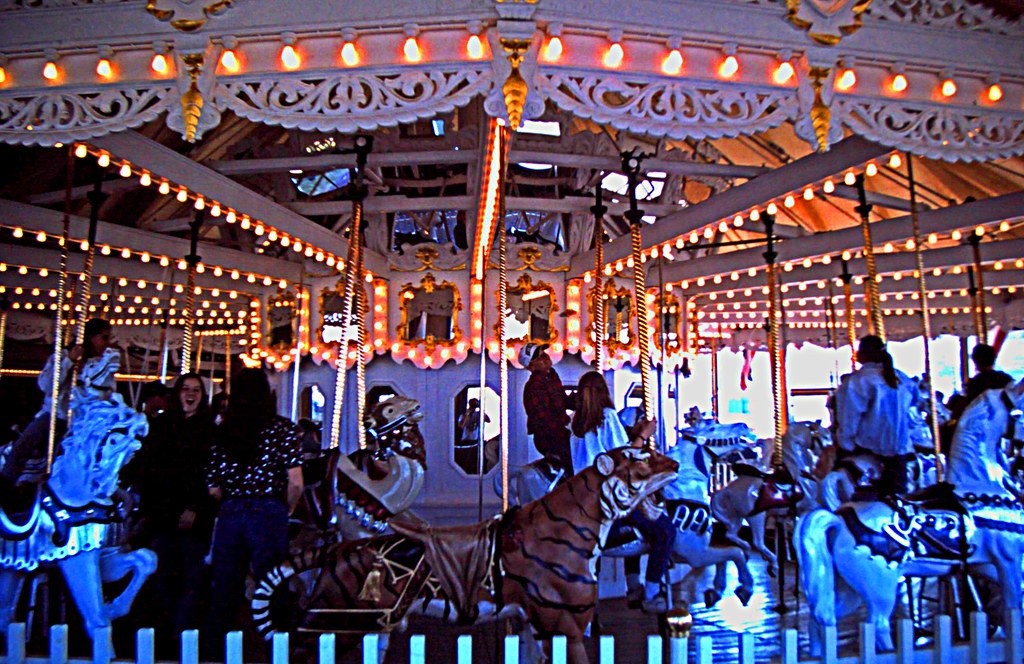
left=834, top=334, right=920, bottom=494
left=457, top=398, right=491, bottom=443
left=517, top=342, right=578, bottom=480
left=0, top=317, right=304, bottom=660
left=966, top=343, right=1014, bottom=403
left=569, top=371, right=689, bottom=613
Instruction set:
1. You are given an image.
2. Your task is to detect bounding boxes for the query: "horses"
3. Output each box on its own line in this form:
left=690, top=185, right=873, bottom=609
left=494, top=378, right=1024, bottom=663
left=79, top=347, right=121, bottom=401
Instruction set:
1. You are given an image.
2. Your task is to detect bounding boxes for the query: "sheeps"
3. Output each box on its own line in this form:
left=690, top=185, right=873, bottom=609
left=336, top=394, right=426, bottom=541
